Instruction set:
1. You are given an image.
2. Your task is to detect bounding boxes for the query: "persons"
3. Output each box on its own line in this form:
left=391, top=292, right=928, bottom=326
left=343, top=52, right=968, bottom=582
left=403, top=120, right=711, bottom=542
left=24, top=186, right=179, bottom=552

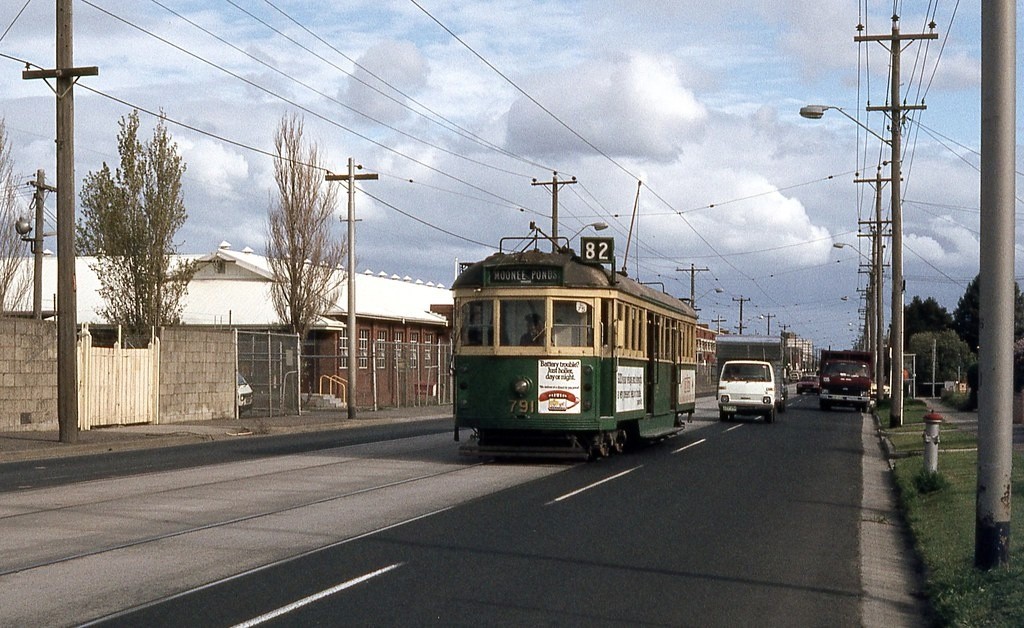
left=726, top=367, right=740, bottom=380
left=903, top=369, right=909, bottom=395
left=520, top=314, right=544, bottom=346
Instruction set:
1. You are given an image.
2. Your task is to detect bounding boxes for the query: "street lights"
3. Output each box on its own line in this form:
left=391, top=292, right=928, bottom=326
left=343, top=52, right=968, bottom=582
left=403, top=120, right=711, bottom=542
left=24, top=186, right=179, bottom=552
left=739, top=315, right=764, bottom=335
left=834, top=241, right=884, bottom=403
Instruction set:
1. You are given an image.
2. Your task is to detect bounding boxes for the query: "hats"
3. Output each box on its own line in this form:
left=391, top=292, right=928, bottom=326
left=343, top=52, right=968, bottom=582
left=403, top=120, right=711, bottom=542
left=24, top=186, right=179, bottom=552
left=525, top=313, right=542, bottom=322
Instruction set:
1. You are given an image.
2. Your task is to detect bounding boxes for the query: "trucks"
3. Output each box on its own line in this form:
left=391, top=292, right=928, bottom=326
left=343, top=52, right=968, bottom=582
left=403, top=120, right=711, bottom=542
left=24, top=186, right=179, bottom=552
left=715, top=359, right=789, bottom=425
left=818, top=345, right=874, bottom=413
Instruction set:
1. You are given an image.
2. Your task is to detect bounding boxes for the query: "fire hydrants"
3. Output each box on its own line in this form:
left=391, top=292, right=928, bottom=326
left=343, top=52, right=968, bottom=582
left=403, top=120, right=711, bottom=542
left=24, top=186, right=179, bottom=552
left=921, top=409, right=943, bottom=479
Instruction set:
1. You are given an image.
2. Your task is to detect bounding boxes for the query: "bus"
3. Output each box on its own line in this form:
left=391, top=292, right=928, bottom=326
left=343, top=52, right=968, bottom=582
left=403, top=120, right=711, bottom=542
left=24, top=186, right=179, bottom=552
left=430, top=218, right=699, bottom=464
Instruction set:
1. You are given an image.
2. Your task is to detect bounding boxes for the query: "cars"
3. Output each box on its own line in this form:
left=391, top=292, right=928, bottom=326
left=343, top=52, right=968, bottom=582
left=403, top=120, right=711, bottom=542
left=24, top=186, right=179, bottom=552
left=797, top=375, right=820, bottom=396
left=238, top=372, right=253, bottom=417
left=790, top=372, right=799, bottom=382
